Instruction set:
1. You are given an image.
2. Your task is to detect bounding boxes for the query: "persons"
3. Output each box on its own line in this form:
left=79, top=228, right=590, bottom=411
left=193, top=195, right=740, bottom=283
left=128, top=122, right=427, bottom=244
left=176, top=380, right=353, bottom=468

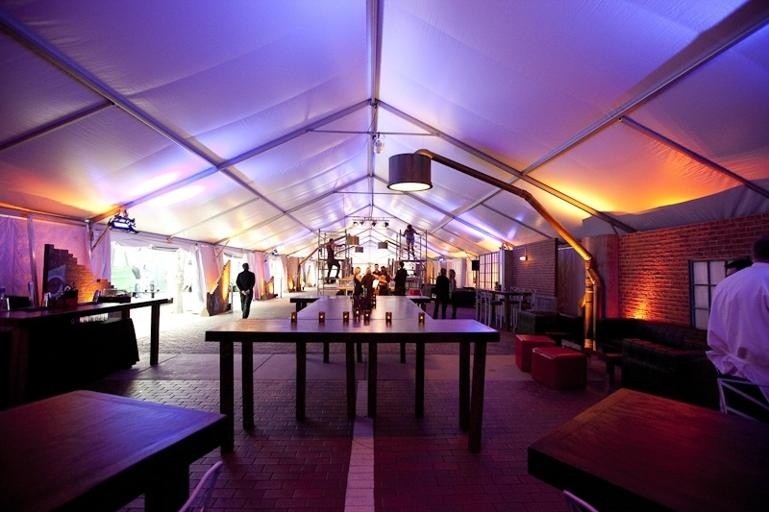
left=351, top=261, right=408, bottom=296
left=724, top=256, right=754, bottom=278
left=399, top=224, right=425, bottom=261
left=705, top=235, right=768, bottom=422
left=428, top=268, right=450, bottom=320
left=323, top=237, right=347, bottom=283
left=448, top=269, right=458, bottom=319
left=236, top=262, right=256, bottom=320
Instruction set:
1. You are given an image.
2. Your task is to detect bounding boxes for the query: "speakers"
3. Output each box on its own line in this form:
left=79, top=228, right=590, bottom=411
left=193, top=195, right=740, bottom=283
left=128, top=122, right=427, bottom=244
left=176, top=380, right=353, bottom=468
left=472, top=260, right=480, bottom=270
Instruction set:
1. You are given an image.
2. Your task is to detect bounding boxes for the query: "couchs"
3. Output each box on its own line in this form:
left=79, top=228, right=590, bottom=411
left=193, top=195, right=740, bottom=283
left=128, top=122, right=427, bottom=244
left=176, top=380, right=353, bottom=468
left=620, top=335, right=717, bottom=405
left=531, top=344, right=590, bottom=392
left=593, top=316, right=712, bottom=364
left=512, top=334, right=555, bottom=372
left=514, top=307, right=584, bottom=344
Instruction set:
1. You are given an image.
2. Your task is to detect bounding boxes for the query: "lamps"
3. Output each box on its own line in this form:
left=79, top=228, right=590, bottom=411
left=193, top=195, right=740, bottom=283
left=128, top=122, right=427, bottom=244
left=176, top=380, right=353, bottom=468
left=345, top=214, right=393, bottom=254
left=106, top=205, right=138, bottom=234
left=386, top=147, right=607, bottom=351
left=367, top=101, right=385, bottom=156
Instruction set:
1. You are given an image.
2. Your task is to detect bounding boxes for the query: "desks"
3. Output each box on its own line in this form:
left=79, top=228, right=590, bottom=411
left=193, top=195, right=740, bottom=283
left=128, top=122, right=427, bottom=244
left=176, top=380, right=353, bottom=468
left=1, top=297, right=177, bottom=403
left=0, top=386, right=231, bottom=512
left=525, top=386, right=764, bottom=511
left=288, top=287, right=440, bottom=425
left=204, top=318, right=503, bottom=457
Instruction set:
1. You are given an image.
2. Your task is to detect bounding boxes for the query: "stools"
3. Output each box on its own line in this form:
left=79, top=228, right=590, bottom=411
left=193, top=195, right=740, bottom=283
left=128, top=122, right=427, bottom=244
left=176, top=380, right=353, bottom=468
left=477, top=288, right=535, bottom=329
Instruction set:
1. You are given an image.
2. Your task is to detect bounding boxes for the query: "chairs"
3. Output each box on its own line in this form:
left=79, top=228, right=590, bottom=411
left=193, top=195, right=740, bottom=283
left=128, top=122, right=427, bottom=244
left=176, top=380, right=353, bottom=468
left=559, top=487, right=603, bottom=511
left=172, top=460, right=226, bottom=512
